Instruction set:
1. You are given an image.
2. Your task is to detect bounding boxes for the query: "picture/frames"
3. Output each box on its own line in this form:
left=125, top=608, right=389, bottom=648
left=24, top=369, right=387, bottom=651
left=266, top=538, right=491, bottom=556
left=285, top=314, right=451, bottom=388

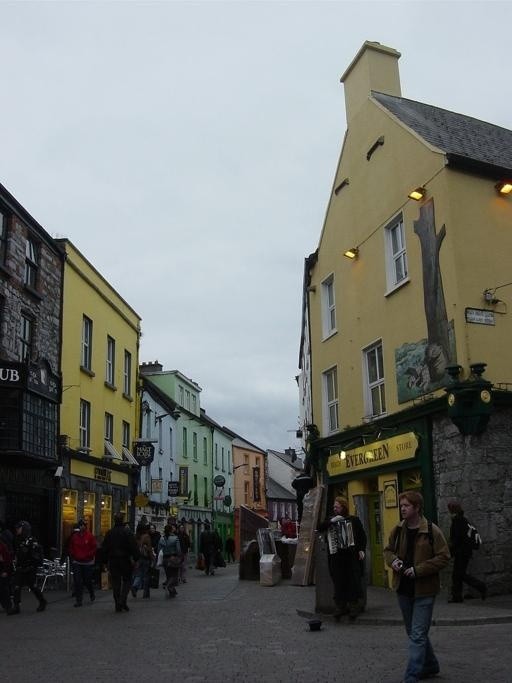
left=384, top=479, right=398, bottom=509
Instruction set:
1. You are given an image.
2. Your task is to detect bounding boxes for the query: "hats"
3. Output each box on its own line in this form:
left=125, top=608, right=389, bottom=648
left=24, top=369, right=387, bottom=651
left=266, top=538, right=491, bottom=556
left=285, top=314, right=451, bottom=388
left=305, top=619, right=324, bottom=631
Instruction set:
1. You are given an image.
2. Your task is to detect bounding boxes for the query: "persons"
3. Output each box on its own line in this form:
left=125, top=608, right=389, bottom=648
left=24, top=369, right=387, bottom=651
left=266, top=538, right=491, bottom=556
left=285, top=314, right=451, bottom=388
left=0, top=515, right=297, bottom=616
left=317, top=496, right=368, bottom=619
left=447, top=501, right=488, bottom=604
left=383, top=490, right=450, bottom=683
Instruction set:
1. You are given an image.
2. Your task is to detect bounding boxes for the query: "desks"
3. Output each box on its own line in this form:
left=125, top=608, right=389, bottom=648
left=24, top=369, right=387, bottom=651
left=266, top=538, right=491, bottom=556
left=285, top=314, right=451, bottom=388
left=34, top=572, right=74, bottom=592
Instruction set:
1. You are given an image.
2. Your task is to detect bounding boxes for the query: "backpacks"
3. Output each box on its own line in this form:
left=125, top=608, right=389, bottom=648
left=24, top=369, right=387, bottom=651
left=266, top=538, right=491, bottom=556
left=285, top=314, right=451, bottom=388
left=453, top=514, right=482, bottom=550
left=25, top=537, right=44, bottom=567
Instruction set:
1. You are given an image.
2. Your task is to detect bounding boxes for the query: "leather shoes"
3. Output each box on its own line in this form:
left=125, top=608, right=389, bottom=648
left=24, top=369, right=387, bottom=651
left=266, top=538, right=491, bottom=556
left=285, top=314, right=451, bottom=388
left=7, top=608, right=20, bottom=616
left=480, top=585, right=488, bottom=601
left=447, top=598, right=463, bottom=603
left=37, top=601, right=47, bottom=609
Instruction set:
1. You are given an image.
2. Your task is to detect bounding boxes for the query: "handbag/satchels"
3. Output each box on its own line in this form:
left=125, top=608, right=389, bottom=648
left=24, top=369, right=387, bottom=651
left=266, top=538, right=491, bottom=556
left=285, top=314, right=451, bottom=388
left=144, top=559, right=160, bottom=589
left=195, top=552, right=205, bottom=571
left=140, top=544, right=158, bottom=558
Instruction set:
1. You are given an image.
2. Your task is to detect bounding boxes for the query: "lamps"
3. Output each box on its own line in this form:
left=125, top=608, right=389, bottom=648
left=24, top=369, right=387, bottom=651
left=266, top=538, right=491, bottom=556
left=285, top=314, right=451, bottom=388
left=344, top=247, right=359, bottom=259
left=338, top=424, right=400, bottom=460
left=495, top=180, right=512, bottom=194
left=408, top=185, right=426, bottom=202
